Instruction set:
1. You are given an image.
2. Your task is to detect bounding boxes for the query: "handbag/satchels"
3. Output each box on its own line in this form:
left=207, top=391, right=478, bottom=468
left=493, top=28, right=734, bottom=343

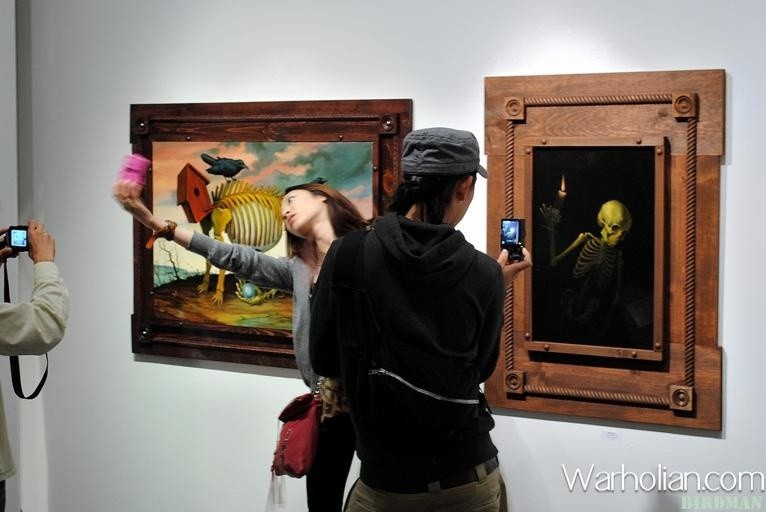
left=269, top=392, right=320, bottom=478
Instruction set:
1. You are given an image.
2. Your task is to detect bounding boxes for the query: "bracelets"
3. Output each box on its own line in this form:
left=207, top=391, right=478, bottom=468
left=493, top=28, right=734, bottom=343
left=145, top=219, right=177, bottom=250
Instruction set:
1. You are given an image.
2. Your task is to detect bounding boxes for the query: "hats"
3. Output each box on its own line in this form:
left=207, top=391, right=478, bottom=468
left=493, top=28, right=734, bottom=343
left=400, top=127, right=489, bottom=179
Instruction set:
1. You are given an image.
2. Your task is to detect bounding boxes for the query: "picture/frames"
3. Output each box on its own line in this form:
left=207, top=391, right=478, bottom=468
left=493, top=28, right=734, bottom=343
left=128, top=98, right=414, bottom=368
left=483, top=68, right=729, bottom=434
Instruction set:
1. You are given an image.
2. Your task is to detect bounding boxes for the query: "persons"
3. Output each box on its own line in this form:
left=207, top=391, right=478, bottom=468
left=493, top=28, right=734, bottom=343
left=111, top=179, right=369, bottom=511
left=308, top=127, right=532, bottom=511
left=0, top=219, right=68, bottom=512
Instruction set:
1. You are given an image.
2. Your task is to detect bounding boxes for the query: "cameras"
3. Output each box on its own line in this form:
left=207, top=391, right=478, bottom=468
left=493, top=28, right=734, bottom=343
left=119, top=153, right=150, bottom=195
left=7, top=225, right=30, bottom=253
left=499, top=218, right=525, bottom=261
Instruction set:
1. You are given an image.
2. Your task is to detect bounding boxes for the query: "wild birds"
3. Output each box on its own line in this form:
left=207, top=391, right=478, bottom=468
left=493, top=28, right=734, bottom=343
left=201, top=153, right=249, bottom=184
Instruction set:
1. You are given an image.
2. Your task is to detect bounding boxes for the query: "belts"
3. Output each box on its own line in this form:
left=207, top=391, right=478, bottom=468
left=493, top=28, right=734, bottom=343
left=360, top=455, right=498, bottom=495
